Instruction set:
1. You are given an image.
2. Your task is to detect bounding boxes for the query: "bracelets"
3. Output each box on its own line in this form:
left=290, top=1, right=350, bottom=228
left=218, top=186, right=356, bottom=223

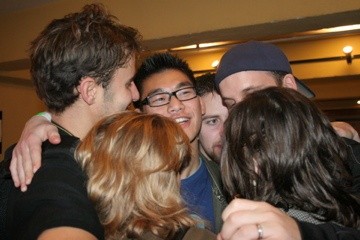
left=35, top=111, right=52, bottom=122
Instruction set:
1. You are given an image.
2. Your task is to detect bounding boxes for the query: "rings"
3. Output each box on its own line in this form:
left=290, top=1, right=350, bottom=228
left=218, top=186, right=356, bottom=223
left=256, top=223, right=263, bottom=239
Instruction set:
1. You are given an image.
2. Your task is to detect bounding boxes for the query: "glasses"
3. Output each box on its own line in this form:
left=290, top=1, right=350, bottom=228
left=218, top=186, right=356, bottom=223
left=139, top=86, right=198, bottom=107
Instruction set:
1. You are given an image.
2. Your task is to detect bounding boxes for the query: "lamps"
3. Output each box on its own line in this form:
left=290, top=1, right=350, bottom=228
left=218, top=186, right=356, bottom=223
left=343, top=46, right=353, bottom=65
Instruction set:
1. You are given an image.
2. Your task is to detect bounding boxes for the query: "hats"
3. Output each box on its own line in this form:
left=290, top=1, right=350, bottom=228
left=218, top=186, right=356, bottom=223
left=215, top=41, right=316, bottom=98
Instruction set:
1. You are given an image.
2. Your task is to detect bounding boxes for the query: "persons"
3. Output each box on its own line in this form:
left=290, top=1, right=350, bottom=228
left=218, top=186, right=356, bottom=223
left=0, top=3, right=360, bottom=240
